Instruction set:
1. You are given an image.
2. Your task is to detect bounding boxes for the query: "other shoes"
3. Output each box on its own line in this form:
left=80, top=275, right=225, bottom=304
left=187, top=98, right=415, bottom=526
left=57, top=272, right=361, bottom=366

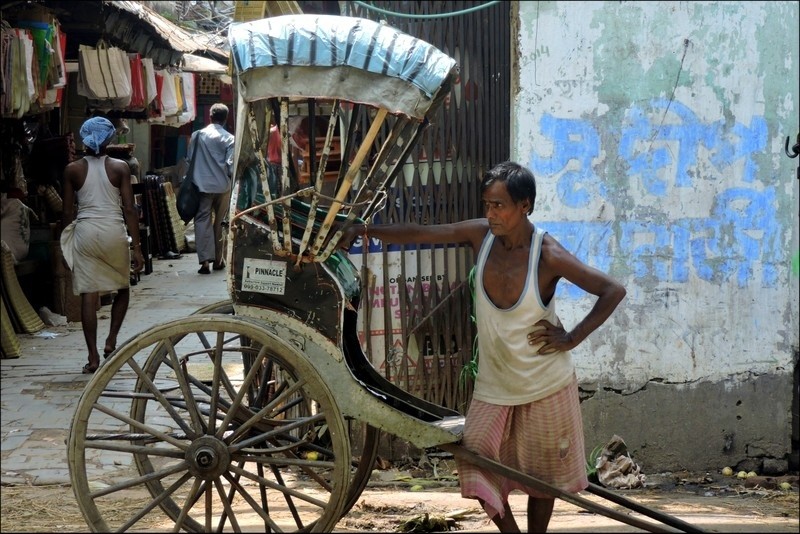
left=213, top=262, right=224, bottom=270
left=198, top=266, right=210, bottom=274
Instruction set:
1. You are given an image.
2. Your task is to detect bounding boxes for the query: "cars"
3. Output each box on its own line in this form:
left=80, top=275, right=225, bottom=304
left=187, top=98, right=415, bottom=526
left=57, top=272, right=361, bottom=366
left=292, top=112, right=341, bottom=162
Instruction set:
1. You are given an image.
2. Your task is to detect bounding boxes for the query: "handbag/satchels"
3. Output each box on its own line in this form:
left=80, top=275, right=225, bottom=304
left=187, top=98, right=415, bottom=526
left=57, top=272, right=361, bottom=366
left=176, top=182, right=200, bottom=226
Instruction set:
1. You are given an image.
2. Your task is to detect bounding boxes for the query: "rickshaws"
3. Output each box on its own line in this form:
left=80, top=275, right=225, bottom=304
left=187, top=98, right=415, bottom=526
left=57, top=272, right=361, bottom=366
left=64, top=15, right=711, bottom=534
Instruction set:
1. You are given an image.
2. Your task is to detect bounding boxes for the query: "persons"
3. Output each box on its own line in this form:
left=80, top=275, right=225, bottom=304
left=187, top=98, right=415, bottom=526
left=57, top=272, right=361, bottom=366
left=347, top=162, right=627, bottom=533
left=61, top=117, right=144, bottom=374
left=184, top=102, right=235, bottom=274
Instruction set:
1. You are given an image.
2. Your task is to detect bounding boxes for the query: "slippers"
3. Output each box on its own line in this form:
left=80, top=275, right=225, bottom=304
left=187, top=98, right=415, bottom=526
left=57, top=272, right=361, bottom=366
left=82, top=363, right=97, bottom=373
left=103, top=353, right=111, bottom=359
